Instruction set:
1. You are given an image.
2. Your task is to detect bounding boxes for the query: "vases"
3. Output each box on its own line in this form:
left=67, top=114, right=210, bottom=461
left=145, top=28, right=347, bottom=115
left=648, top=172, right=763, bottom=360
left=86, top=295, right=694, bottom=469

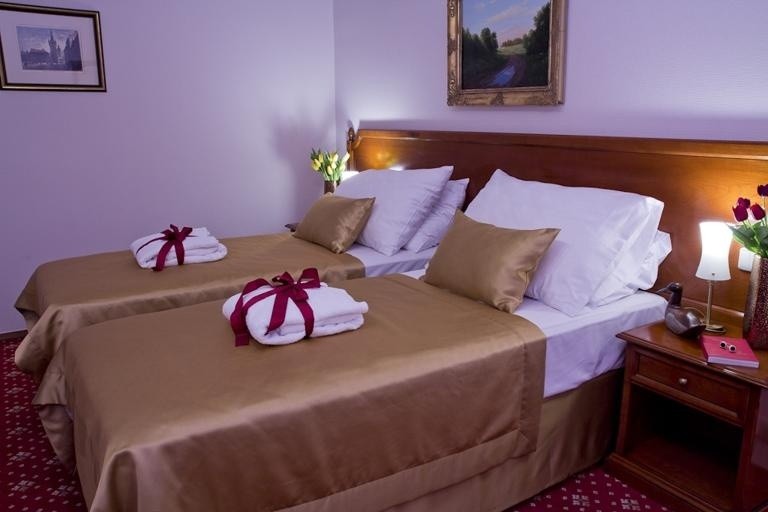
left=742, top=254, right=767, bottom=352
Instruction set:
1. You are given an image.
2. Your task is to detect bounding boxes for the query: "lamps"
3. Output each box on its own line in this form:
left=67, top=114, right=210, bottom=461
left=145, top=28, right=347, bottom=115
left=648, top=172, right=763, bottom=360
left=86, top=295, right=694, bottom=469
left=694, top=220, right=733, bottom=333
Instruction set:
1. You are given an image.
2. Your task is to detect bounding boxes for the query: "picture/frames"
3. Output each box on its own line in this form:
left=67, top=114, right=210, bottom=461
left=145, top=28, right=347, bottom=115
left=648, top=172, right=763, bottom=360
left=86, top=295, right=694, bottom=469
left=445, top=1, right=570, bottom=108
left=0, top=2, right=108, bottom=93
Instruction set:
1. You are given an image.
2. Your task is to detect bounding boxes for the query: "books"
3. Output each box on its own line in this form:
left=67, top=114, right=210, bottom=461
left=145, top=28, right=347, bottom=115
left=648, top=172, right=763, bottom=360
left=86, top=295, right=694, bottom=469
left=701, top=334, right=760, bottom=369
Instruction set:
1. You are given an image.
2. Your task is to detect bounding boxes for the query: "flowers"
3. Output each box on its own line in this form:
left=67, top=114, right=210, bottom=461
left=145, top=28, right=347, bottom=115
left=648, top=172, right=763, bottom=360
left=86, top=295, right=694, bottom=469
left=724, top=182, right=767, bottom=256
left=308, top=146, right=350, bottom=181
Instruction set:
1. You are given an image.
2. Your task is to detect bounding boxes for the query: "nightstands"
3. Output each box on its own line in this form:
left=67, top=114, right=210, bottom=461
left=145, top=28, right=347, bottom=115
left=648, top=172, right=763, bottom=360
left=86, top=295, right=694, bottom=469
left=602, top=321, right=768, bottom=512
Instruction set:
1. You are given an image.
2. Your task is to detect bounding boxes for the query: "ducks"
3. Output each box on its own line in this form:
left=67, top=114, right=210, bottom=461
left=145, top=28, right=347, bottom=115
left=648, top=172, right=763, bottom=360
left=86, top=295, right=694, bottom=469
left=655, top=281, right=706, bottom=338
